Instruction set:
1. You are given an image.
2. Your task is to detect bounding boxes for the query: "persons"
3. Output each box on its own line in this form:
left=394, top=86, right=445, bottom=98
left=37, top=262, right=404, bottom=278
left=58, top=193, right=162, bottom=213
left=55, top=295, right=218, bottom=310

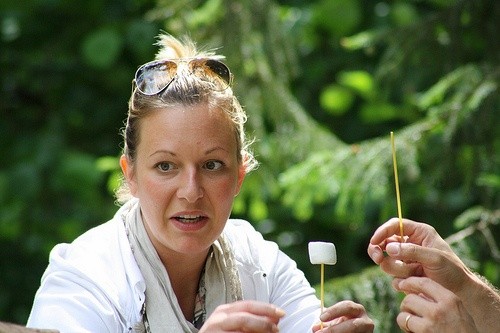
left=26, top=33, right=375, bottom=333
left=367, top=217, right=500, bottom=333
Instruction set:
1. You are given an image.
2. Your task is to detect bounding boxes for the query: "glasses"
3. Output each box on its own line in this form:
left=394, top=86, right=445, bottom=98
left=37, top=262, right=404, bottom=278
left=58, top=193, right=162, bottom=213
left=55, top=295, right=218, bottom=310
left=132, top=58, right=233, bottom=95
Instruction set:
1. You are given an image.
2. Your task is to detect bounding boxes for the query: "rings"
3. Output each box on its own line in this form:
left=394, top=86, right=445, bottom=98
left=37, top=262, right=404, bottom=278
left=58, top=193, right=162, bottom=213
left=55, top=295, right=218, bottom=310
left=405, top=314, right=412, bottom=330
